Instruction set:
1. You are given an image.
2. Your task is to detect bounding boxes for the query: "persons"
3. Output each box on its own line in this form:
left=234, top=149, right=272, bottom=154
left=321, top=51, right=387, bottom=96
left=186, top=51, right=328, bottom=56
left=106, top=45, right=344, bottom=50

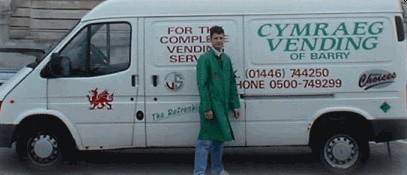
left=193, top=26, right=241, bottom=175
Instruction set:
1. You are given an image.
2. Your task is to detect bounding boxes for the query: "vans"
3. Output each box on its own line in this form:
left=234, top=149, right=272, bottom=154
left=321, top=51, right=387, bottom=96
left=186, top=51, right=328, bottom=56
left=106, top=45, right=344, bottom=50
left=0, top=42, right=49, bottom=86
left=0, top=0, right=407, bottom=175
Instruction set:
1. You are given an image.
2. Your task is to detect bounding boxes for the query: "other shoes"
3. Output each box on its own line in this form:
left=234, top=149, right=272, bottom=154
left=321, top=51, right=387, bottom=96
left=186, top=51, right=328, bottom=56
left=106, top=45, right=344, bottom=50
left=220, top=170, right=229, bottom=175
